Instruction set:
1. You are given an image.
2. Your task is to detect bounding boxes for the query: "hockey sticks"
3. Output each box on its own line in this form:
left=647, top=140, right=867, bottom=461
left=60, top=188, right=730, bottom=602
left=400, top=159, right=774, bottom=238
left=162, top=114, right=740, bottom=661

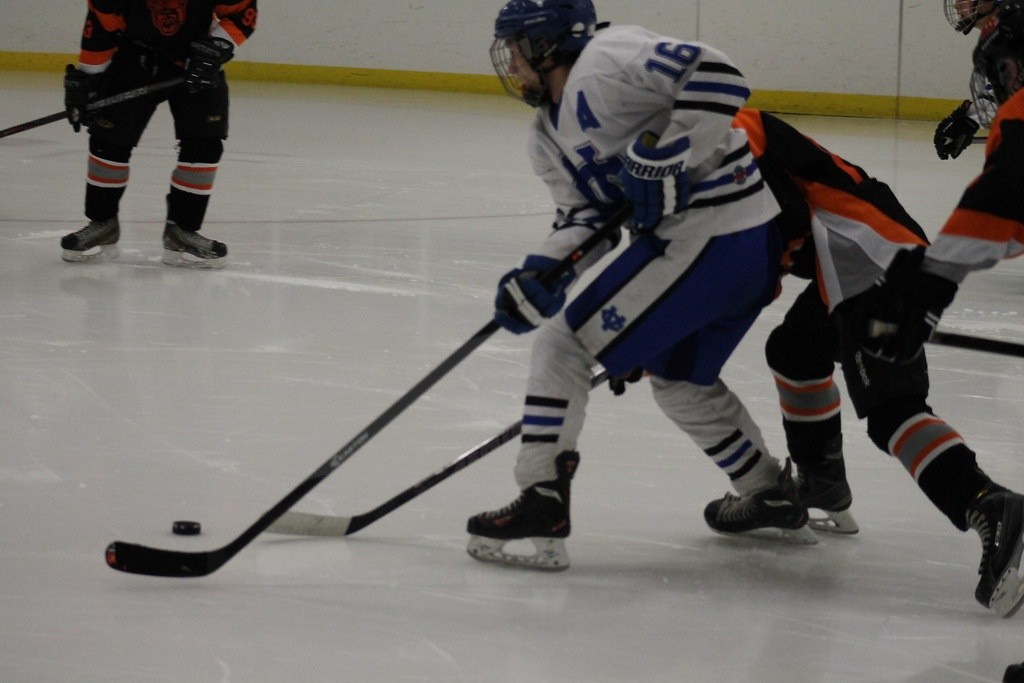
left=264, top=369, right=611, bottom=537
left=0, top=77, right=187, bottom=138
left=104, top=148, right=725, bottom=579
left=929, top=331, right=1024, bottom=358
left=942, top=136, right=987, bottom=145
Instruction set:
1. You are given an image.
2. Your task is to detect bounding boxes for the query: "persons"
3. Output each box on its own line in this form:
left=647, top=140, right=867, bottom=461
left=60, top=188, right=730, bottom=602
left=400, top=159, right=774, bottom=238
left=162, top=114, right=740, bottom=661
left=62, top=0, right=258, bottom=269
left=466, top=0, right=822, bottom=571
left=732, top=109, right=1024, bottom=617
left=853, top=0, right=1024, bottom=683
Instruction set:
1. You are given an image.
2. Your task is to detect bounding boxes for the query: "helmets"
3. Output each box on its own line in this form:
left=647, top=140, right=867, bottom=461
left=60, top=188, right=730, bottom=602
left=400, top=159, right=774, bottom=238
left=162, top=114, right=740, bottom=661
left=494, top=0, right=597, bottom=108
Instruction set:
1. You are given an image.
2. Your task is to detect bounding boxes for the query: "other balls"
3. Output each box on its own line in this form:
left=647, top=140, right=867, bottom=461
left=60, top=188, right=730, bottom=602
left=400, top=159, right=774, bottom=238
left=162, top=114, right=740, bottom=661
left=172, top=521, right=201, bottom=536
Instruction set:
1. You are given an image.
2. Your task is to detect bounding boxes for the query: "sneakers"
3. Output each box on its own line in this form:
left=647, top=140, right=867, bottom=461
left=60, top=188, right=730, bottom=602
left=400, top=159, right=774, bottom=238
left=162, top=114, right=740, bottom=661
left=466, top=450, right=580, bottom=569
left=704, top=457, right=819, bottom=549
left=60, top=213, right=122, bottom=262
left=792, top=432, right=858, bottom=533
left=966, top=468, right=1024, bottom=618
left=160, top=193, right=227, bottom=268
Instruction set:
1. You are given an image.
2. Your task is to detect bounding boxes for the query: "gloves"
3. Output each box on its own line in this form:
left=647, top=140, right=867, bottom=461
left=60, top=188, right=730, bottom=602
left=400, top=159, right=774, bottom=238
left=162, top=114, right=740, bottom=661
left=607, top=131, right=691, bottom=236
left=64, top=64, right=115, bottom=133
left=608, top=365, right=643, bottom=395
left=183, top=38, right=234, bottom=111
left=494, top=255, right=578, bottom=334
left=934, top=99, right=980, bottom=160
left=858, top=246, right=958, bottom=366
